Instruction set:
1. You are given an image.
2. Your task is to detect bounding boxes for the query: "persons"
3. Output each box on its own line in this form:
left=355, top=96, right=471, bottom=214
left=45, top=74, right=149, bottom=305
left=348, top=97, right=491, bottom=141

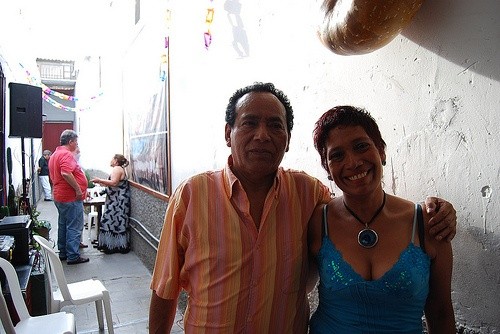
left=48, top=128, right=89, bottom=265
left=37, top=149, right=53, bottom=201
left=305, top=105, right=457, bottom=334
left=89, top=154, right=131, bottom=254
left=148, top=81, right=457, bottom=334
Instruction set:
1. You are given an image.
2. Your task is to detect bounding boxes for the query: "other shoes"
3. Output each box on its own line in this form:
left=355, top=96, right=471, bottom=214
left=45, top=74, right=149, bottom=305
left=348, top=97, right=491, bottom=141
left=59, top=256, right=89, bottom=264
left=79, top=242, right=88, bottom=248
left=44, top=198, right=52, bottom=201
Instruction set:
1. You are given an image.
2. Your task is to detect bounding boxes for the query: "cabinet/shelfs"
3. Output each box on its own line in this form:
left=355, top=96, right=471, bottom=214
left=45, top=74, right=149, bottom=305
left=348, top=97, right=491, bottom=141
left=3, top=255, right=52, bottom=327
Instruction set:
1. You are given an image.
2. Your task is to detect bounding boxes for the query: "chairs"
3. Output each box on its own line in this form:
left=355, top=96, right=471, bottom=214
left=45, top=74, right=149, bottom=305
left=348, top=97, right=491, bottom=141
left=0, top=257, right=77, bottom=334
left=33, top=235, right=114, bottom=334
left=84, top=189, right=104, bottom=235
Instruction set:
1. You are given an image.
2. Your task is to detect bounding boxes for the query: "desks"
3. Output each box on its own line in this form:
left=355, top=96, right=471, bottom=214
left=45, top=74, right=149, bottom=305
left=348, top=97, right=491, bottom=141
left=83, top=197, right=106, bottom=234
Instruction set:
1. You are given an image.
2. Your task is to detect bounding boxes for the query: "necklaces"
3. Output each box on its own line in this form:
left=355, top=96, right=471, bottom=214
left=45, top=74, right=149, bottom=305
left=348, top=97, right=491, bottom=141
left=343, top=190, right=386, bottom=249
